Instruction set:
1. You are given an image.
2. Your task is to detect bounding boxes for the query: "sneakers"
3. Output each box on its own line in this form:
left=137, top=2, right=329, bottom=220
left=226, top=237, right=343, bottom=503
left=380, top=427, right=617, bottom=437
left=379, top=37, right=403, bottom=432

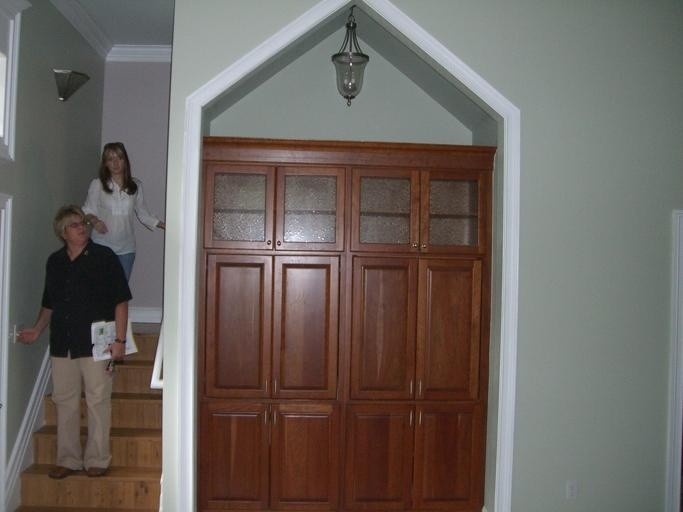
left=87, top=466, right=106, bottom=476
left=49, top=466, right=71, bottom=479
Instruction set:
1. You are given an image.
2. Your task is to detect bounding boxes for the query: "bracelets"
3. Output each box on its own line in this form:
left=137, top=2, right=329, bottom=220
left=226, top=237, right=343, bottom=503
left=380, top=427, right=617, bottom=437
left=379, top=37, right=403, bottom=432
left=115, top=339, right=126, bottom=344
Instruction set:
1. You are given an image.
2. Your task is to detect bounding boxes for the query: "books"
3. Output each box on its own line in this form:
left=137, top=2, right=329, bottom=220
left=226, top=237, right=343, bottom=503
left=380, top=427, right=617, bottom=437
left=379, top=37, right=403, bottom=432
left=90, top=321, right=138, bottom=362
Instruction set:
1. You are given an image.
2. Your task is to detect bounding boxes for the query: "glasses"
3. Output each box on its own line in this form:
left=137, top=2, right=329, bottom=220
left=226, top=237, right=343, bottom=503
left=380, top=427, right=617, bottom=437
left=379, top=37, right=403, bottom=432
left=68, top=220, right=86, bottom=228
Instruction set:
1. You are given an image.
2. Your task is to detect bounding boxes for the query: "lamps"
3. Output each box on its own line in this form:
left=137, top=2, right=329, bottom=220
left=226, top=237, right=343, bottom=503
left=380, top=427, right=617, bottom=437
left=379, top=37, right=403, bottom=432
left=50, top=68, right=91, bottom=100
left=330, top=4, right=371, bottom=105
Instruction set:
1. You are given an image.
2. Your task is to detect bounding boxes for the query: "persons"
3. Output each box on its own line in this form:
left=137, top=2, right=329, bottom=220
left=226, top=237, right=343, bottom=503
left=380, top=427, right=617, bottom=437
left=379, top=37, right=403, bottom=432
left=81, top=142, right=165, bottom=283
left=18, top=205, right=133, bottom=480
